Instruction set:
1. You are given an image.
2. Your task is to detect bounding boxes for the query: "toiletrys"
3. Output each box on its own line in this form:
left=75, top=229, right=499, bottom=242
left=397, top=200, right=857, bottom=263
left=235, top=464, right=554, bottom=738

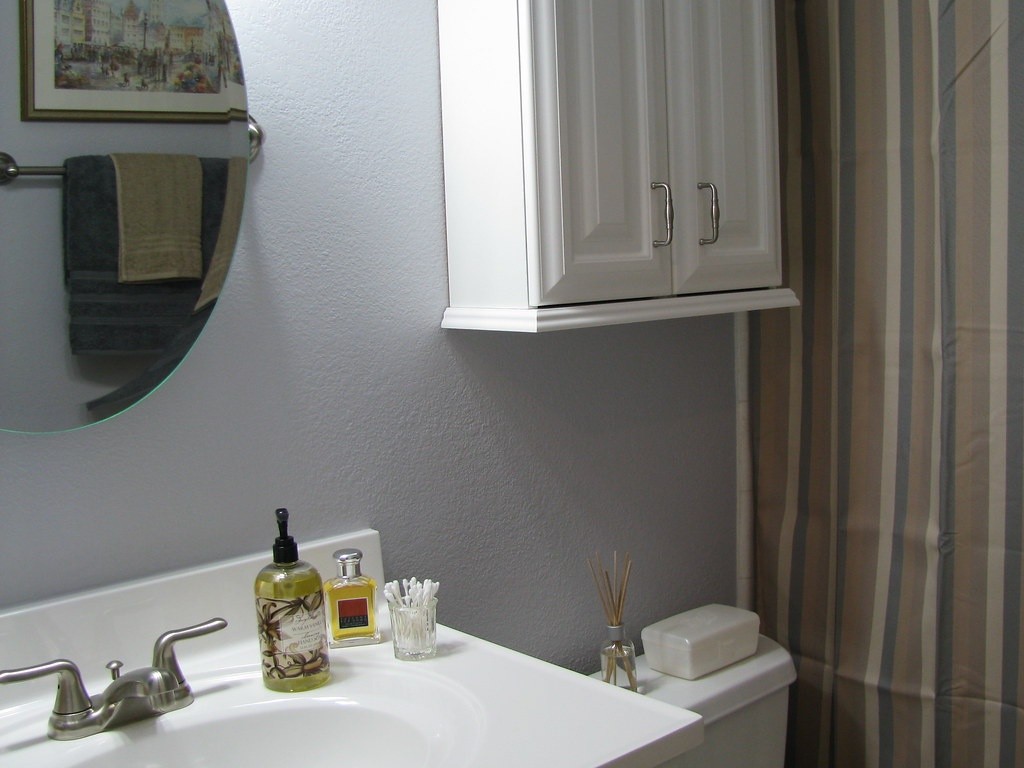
left=253, top=507, right=332, bottom=693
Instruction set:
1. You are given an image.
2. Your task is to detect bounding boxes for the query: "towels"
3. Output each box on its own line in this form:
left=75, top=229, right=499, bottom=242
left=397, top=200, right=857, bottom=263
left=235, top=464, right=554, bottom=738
left=61, top=151, right=248, bottom=419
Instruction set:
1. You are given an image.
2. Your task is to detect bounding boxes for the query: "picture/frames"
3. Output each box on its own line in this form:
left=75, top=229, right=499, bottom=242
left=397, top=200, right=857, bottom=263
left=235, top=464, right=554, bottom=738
left=19, top=0, right=249, bottom=125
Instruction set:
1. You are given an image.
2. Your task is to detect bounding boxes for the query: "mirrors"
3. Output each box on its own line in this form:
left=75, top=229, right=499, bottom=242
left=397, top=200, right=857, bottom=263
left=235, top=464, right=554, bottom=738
left=0, top=0, right=249, bottom=435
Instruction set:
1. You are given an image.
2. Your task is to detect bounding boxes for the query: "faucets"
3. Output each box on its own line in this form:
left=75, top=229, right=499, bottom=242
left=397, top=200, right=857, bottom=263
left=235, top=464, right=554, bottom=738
left=1, top=616, right=228, bottom=742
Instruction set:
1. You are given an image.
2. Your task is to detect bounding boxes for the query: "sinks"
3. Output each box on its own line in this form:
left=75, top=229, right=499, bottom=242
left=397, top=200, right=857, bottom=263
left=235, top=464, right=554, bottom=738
left=1, top=662, right=492, bottom=767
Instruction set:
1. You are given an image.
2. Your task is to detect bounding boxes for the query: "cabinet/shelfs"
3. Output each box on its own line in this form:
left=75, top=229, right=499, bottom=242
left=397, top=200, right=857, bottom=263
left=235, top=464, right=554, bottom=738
left=436, top=0, right=802, bottom=334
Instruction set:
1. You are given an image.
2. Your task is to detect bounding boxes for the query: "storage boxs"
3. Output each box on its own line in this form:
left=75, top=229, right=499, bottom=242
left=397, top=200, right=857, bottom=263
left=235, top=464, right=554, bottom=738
left=640, top=603, right=761, bottom=681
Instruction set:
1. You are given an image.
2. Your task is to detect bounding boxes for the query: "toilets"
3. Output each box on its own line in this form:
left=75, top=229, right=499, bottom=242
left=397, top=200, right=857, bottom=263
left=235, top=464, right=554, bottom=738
left=586, top=633, right=797, bottom=768
